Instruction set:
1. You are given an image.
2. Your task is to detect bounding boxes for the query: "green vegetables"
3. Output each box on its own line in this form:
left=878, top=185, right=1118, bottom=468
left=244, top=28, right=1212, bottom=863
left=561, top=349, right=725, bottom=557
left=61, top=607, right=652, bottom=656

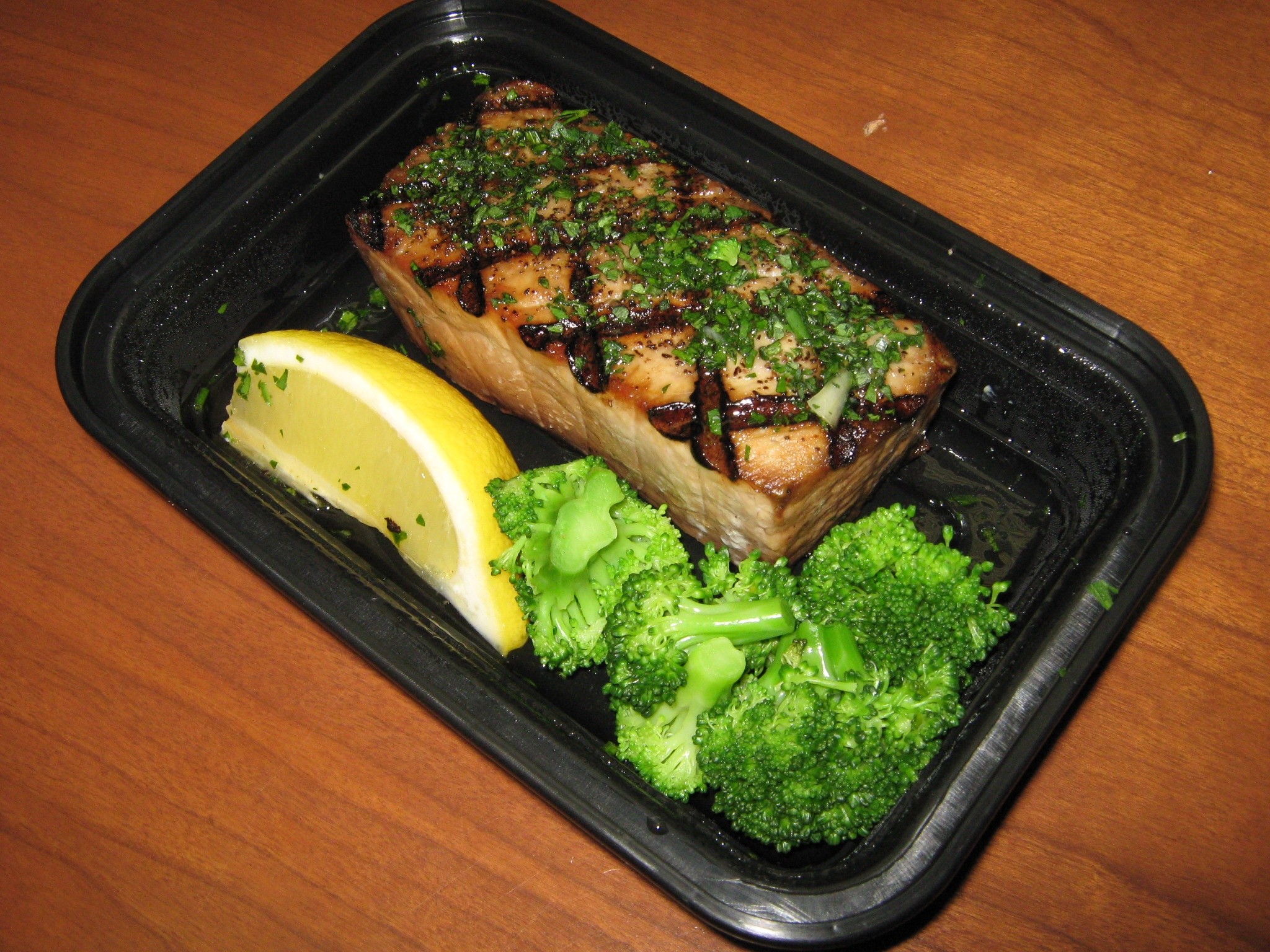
left=191, top=73, right=930, bottom=548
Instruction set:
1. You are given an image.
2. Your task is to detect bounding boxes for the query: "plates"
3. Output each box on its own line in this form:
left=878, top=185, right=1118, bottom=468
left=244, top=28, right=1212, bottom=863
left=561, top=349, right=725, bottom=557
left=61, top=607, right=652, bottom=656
left=53, top=0, right=1214, bottom=952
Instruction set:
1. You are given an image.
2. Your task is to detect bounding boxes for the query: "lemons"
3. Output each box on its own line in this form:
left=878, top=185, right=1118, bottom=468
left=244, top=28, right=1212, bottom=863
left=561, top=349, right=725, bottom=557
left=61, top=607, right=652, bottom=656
left=221, top=327, right=534, bottom=660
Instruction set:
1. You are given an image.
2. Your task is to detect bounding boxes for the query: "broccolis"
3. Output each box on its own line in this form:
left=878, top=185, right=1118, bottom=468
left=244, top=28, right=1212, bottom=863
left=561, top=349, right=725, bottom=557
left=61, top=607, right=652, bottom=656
left=486, top=455, right=1017, bottom=854
